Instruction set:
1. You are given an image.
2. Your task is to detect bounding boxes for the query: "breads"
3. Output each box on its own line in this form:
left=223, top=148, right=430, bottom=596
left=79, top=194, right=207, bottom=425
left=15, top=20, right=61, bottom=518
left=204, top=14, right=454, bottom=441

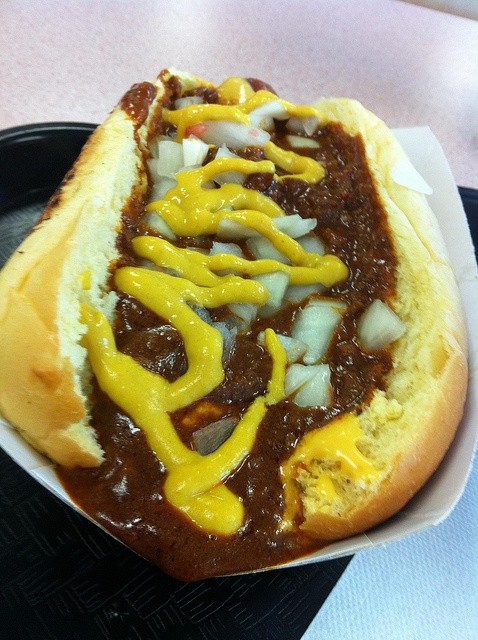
left=0, top=66, right=469, bottom=578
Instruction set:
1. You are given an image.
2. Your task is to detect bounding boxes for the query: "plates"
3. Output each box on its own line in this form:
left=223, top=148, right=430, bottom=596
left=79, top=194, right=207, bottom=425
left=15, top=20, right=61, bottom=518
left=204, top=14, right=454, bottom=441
left=1, top=121, right=478, bottom=639
